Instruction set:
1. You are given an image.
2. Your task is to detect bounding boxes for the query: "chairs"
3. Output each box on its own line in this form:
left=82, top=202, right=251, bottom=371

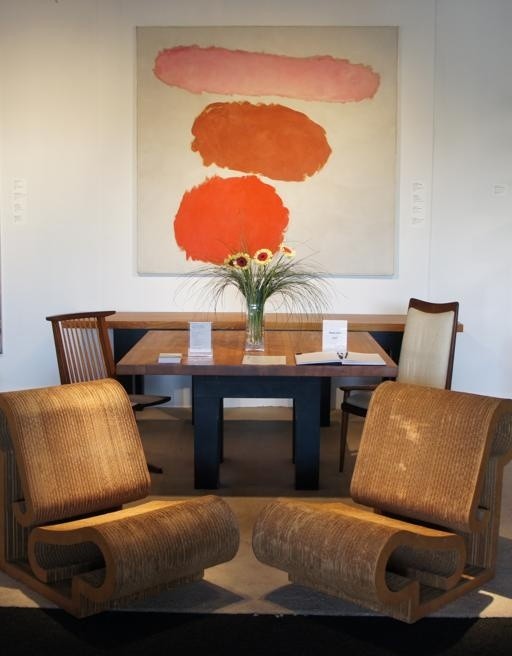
left=46, top=309, right=171, bottom=474
left=1, top=379, right=240, bottom=618
left=337, top=300, right=462, bottom=472
left=253, top=383, right=510, bottom=624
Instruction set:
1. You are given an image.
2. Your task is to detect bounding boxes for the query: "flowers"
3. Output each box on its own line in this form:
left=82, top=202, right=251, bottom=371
left=192, top=249, right=338, bottom=336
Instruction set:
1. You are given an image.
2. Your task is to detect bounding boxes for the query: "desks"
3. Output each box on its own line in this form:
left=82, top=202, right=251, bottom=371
left=66, top=310, right=465, bottom=425
left=116, top=327, right=395, bottom=493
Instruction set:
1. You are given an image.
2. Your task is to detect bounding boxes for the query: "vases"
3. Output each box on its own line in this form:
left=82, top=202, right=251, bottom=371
left=246, top=304, right=266, bottom=352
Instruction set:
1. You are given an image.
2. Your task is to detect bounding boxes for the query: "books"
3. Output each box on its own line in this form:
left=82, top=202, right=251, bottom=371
left=294, top=351, right=387, bottom=366
